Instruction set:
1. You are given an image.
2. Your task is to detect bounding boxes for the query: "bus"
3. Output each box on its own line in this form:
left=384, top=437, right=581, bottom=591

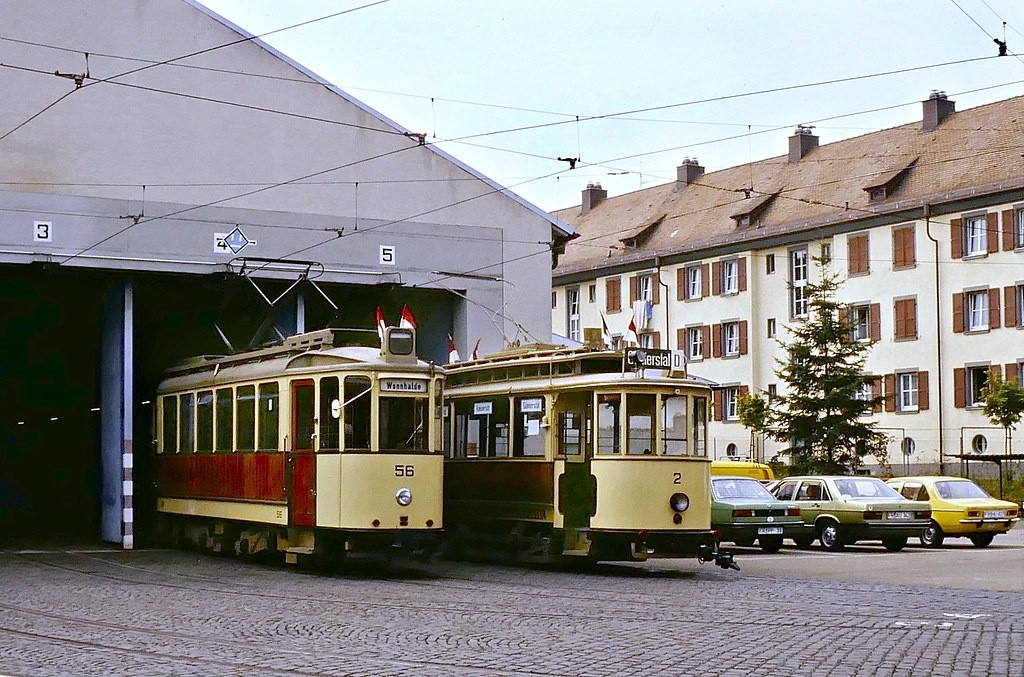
left=151, top=256, right=444, bottom=573
left=413, top=268, right=742, bottom=571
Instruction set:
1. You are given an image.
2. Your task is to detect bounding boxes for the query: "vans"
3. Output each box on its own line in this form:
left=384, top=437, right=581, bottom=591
left=708, top=460, right=773, bottom=480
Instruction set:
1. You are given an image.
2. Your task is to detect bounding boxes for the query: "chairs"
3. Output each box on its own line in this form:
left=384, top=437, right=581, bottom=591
left=806, top=485, right=821, bottom=500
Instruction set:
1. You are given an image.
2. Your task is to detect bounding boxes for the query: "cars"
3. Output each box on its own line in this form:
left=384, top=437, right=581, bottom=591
left=709, top=475, right=816, bottom=554
left=873, top=476, right=1021, bottom=548
left=754, top=476, right=931, bottom=552
